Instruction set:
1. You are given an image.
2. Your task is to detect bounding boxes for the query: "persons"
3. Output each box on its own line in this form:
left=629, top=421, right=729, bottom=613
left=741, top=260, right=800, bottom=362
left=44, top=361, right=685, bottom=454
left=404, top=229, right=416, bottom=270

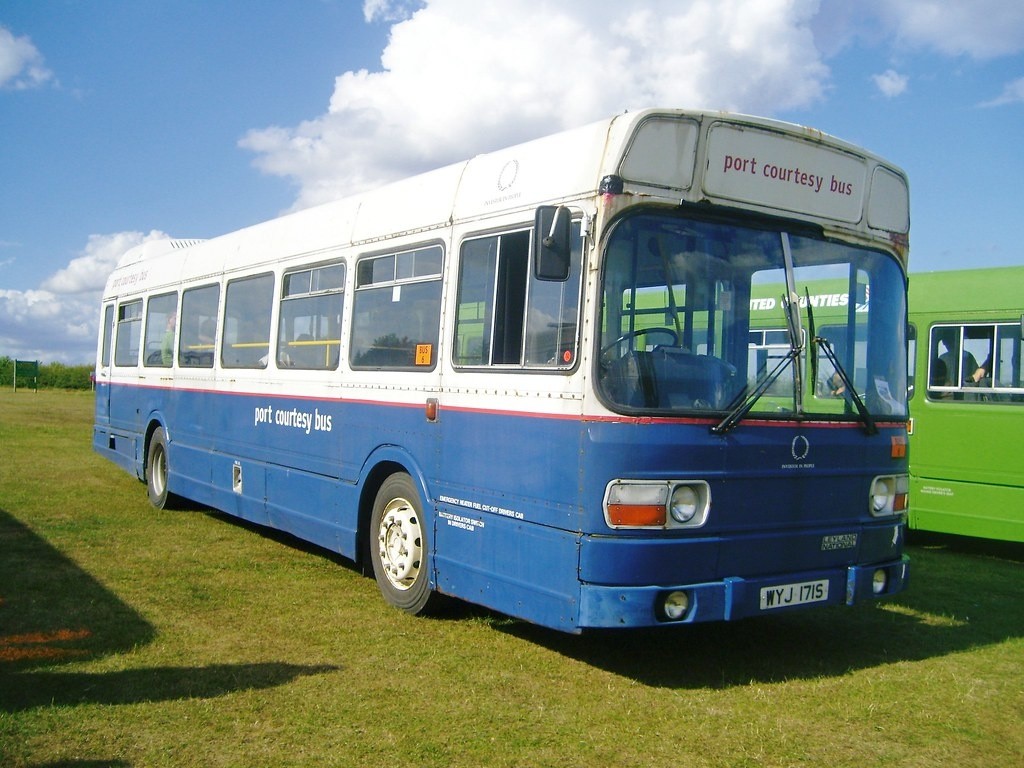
left=159, top=309, right=187, bottom=367
left=939, top=335, right=979, bottom=385
left=185, top=319, right=227, bottom=367
left=829, top=333, right=1013, bottom=402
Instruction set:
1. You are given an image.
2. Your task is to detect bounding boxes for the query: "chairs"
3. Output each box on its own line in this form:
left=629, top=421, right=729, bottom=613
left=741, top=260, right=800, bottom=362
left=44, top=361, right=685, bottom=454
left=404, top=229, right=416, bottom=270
left=979, top=377, right=1012, bottom=401
left=293, top=334, right=315, bottom=368
left=146, top=341, right=164, bottom=364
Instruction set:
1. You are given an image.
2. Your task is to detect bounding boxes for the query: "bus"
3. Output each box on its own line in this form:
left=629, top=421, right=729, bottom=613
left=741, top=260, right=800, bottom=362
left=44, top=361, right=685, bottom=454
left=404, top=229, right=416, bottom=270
left=456, top=264, right=1024, bottom=544
left=92, top=110, right=912, bottom=634
